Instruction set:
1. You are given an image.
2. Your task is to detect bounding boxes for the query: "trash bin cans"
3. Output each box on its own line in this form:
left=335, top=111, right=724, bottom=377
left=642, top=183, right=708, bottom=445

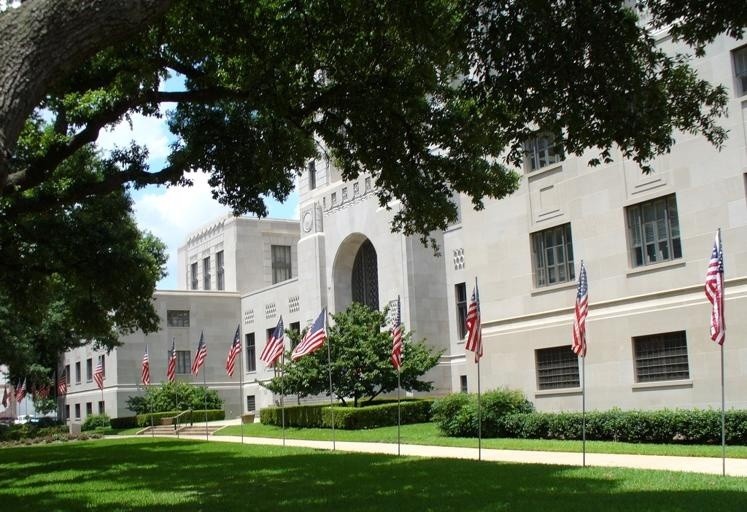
left=241, top=415, right=255, bottom=424
left=70, top=423, right=81, bottom=434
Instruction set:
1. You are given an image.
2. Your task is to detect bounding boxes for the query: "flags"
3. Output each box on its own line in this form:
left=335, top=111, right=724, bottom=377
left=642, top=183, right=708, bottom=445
left=1, top=370, right=68, bottom=408
left=222, top=323, right=243, bottom=378
left=91, top=352, right=105, bottom=390
left=391, top=298, right=406, bottom=372
left=570, top=263, right=592, bottom=357
left=466, top=284, right=486, bottom=364
left=191, top=331, right=208, bottom=374
left=166, top=339, right=177, bottom=384
left=291, top=307, right=327, bottom=361
left=704, top=232, right=731, bottom=346
left=259, top=316, right=286, bottom=370
left=141, top=345, right=151, bottom=388
